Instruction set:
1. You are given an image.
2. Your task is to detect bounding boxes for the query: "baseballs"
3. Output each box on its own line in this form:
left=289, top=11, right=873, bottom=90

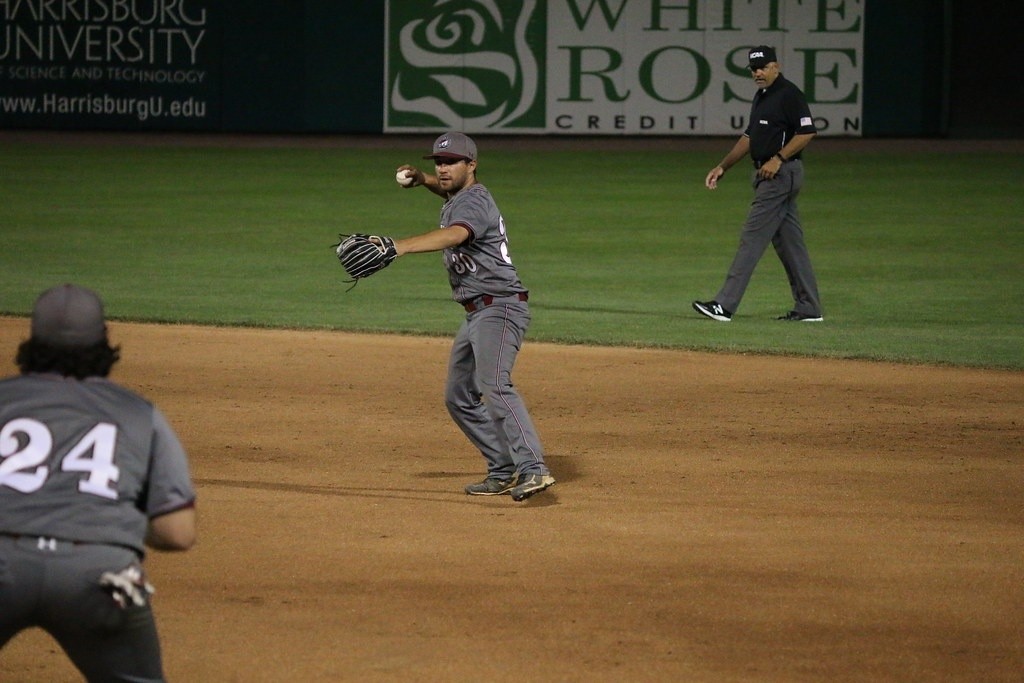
left=396, top=169, right=413, bottom=186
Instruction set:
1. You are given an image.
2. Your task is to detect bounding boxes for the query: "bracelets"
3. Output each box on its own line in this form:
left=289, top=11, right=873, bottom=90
left=776, top=152, right=786, bottom=162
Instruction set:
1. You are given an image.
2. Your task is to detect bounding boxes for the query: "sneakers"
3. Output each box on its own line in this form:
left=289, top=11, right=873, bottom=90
left=778, top=311, right=824, bottom=322
left=466, top=477, right=516, bottom=495
left=693, top=300, right=732, bottom=321
left=511, top=473, right=556, bottom=501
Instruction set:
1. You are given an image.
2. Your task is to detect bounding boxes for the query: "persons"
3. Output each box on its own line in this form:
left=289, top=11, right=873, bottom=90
left=0, top=283, right=197, bottom=683
left=692, top=45, right=824, bottom=322
left=336, top=131, right=557, bottom=502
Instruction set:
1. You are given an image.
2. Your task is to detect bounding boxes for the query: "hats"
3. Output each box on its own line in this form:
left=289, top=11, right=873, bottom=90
left=423, top=132, right=479, bottom=160
left=744, top=45, right=777, bottom=70
left=32, top=284, right=105, bottom=350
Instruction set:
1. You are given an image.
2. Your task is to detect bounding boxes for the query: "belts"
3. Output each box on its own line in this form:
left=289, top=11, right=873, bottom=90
left=755, top=153, right=802, bottom=169
left=465, top=292, right=528, bottom=313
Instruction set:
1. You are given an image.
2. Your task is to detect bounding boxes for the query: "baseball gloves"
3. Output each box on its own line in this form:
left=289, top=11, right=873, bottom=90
left=336, top=233, right=397, bottom=280
